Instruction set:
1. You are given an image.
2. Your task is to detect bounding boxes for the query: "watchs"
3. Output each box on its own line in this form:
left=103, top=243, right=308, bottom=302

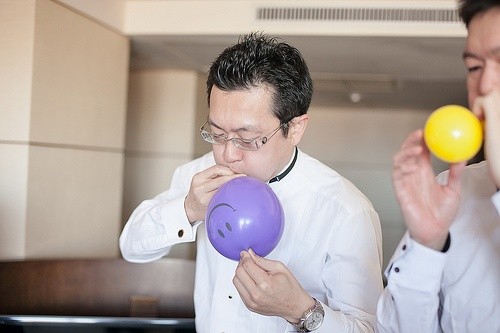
left=290, top=297, right=325, bottom=333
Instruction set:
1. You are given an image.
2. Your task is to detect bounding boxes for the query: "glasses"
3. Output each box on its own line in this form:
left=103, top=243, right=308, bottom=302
left=198, top=114, right=298, bottom=153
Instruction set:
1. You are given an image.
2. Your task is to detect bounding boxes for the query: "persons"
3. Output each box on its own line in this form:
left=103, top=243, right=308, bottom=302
left=119, top=29, right=384, bottom=333
left=375, top=0, right=500, bottom=333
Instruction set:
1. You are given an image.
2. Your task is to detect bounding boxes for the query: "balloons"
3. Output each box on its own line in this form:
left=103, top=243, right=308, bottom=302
left=424, top=105, right=484, bottom=164
left=206, top=175, right=285, bottom=261
